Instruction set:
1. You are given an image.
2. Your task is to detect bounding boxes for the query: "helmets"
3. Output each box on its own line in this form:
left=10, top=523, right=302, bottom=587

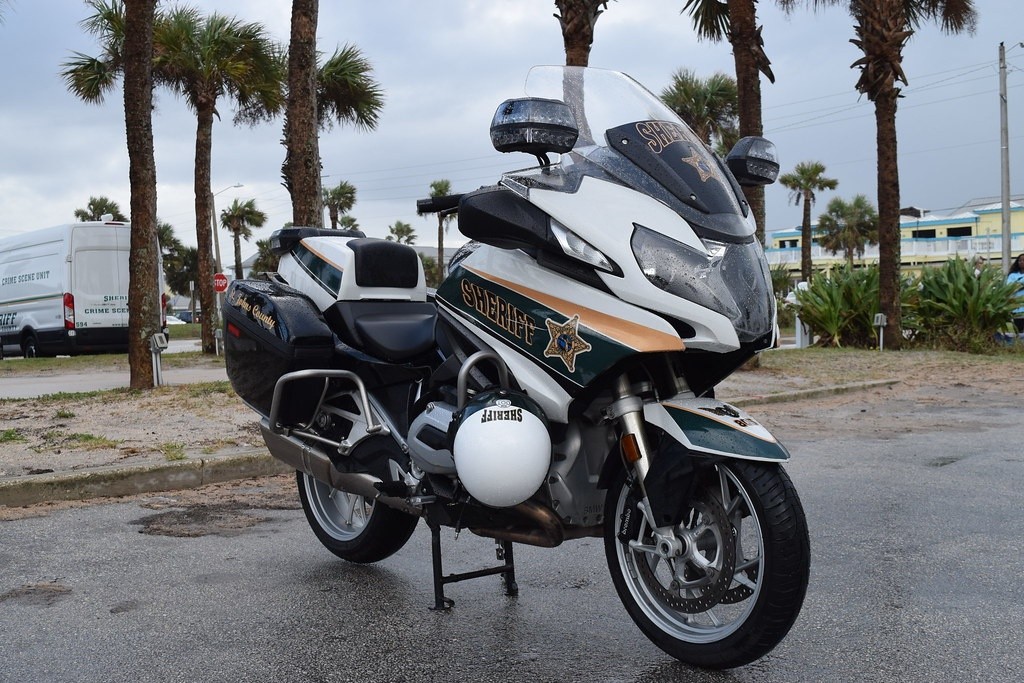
left=449, top=393, right=554, bottom=508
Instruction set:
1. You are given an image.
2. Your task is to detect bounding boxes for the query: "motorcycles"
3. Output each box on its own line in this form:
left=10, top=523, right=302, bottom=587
left=222, top=65, right=813, bottom=671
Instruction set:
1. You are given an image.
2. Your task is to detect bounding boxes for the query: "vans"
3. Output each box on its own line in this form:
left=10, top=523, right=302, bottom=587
left=179, top=311, right=202, bottom=323
left=0, top=221, right=169, bottom=359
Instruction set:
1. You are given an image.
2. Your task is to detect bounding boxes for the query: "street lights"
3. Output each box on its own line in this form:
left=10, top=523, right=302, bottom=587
left=212, top=183, right=244, bottom=307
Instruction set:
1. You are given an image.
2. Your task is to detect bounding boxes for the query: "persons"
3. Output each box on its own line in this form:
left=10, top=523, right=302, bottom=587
left=974, top=256, right=983, bottom=277
left=1004, top=254, right=1024, bottom=334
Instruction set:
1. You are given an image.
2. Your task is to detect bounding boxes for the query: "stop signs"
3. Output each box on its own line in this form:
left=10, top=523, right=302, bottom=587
left=214, top=273, right=228, bottom=292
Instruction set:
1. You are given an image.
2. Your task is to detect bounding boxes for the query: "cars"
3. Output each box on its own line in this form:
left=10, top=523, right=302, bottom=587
left=166, top=316, right=187, bottom=324
